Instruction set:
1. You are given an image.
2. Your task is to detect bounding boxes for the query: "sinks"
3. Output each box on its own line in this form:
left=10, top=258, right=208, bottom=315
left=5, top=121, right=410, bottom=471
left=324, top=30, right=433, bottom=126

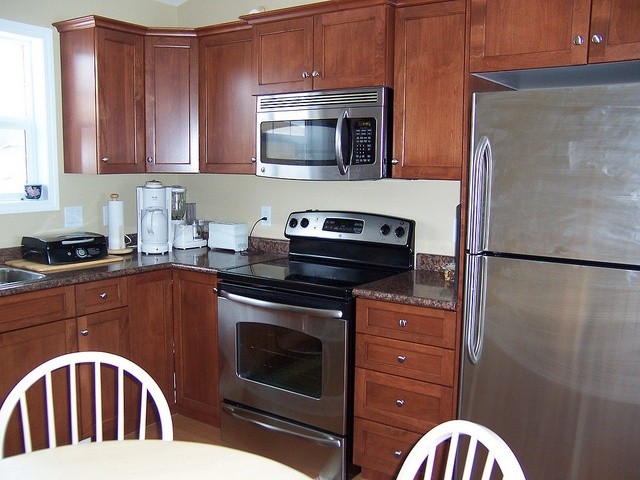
left=0, top=268, right=33, bottom=286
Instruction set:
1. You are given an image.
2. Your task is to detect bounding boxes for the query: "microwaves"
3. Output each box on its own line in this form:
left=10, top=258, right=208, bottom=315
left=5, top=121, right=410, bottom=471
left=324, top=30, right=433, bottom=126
left=255, top=86, right=392, bottom=182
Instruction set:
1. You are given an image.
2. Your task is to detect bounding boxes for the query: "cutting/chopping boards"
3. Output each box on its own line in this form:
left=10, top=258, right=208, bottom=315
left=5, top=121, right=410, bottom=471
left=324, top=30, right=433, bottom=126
left=4, top=253, right=123, bottom=272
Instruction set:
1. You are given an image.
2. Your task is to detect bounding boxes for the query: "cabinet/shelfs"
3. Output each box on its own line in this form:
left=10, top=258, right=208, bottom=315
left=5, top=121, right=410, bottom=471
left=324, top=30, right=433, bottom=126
left=354, top=300, right=457, bottom=479
left=470, top=2, right=640, bottom=74
left=73, top=277, right=130, bottom=439
left=0, top=287, right=84, bottom=455
left=144, top=35, right=199, bottom=173
left=251, top=3, right=387, bottom=95
left=392, top=2, right=468, bottom=179
left=94, top=26, right=145, bottom=174
left=198, top=29, right=256, bottom=174
left=127, top=268, right=172, bottom=434
left=172, top=269, right=220, bottom=430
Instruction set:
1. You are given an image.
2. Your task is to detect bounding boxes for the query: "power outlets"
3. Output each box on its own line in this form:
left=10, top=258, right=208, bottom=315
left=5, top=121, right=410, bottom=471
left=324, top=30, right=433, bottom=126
left=261, top=206, right=273, bottom=226
left=65, top=206, right=84, bottom=228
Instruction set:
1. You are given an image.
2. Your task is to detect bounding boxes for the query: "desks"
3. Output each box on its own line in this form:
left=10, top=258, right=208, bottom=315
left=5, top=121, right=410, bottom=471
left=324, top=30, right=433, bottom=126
left=0, top=439, right=313, bottom=480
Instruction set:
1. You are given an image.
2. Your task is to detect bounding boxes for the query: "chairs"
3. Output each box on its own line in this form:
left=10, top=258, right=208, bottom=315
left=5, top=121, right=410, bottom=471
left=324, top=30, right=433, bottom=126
left=395, top=421, right=525, bottom=480
left=0, top=352, right=175, bottom=458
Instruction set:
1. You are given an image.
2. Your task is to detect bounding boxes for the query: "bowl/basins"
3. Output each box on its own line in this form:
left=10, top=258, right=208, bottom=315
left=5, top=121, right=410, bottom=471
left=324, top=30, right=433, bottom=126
left=23, top=184, right=42, bottom=200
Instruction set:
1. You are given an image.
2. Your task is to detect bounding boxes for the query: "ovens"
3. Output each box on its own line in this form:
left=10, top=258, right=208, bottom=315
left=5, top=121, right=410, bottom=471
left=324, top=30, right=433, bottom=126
left=211, top=280, right=361, bottom=479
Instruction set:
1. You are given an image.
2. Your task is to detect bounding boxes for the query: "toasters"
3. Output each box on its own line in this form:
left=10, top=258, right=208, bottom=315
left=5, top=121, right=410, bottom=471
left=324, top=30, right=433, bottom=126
left=208, top=220, right=249, bottom=252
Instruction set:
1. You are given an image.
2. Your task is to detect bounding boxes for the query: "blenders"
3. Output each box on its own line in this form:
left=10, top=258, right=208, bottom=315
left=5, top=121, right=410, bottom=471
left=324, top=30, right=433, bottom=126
left=174, top=203, right=207, bottom=250
left=172, top=184, right=186, bottom=247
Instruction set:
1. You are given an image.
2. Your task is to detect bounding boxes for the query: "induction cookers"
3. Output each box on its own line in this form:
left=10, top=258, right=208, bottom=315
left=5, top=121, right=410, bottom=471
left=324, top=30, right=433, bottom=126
left=219, top=254, right=405, bottom=295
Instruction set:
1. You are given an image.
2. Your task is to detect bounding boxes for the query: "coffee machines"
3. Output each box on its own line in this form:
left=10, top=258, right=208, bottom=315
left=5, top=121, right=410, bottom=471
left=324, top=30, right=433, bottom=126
left=135, top=180, right=172, bottom=255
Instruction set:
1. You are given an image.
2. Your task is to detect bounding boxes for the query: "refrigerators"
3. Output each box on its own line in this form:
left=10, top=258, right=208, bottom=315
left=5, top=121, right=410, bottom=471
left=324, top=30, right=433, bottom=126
left=455, top=83, right=640, bottom=480
